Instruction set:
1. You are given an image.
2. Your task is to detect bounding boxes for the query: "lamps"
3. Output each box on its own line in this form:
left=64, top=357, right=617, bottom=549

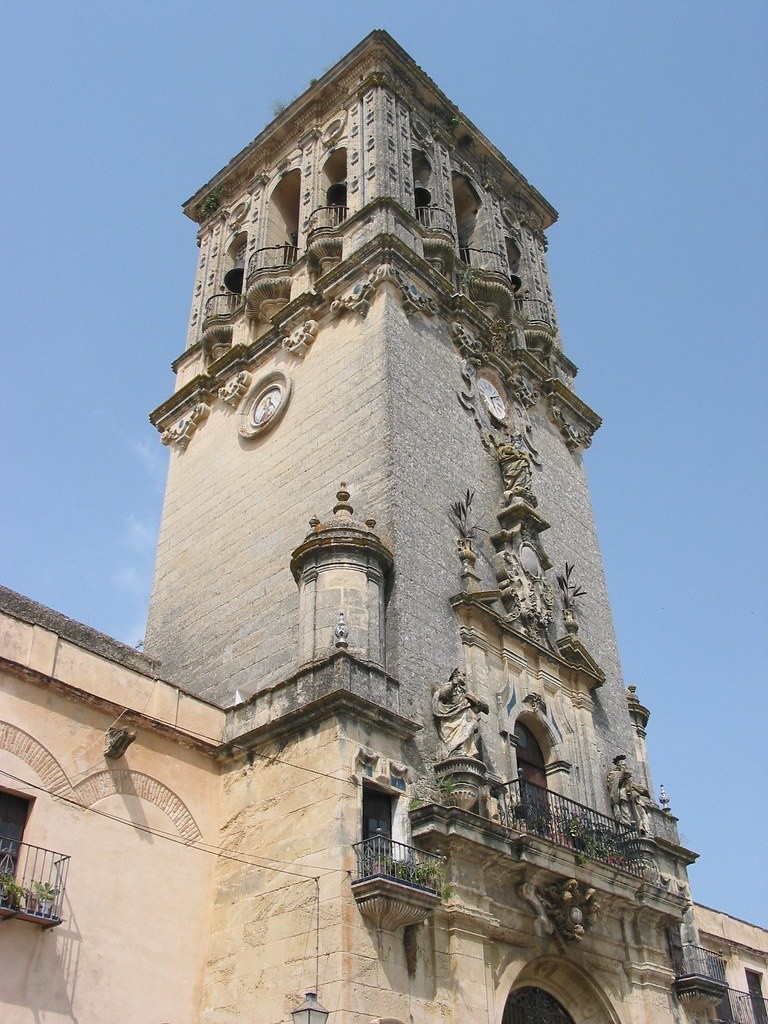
left=292, top=993, right=329, bottom=1023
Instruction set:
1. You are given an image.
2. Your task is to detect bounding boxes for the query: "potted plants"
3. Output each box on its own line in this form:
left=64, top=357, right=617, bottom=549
left=553, top=806, right=622, bottom=865
left=0, top=874, right=61, bottom=915
left=371, top=852, right=454, bottom=903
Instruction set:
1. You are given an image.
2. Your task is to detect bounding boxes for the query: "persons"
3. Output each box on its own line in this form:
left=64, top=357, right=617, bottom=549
left=607, top=755, right=650, bottom=831
left=432, top=667, right=489, bottom=760
left=498, top=443, right=538, bottom=505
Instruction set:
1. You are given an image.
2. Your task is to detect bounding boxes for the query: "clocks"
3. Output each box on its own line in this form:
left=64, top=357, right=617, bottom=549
left=477, top=379, right=506, bottom=419
